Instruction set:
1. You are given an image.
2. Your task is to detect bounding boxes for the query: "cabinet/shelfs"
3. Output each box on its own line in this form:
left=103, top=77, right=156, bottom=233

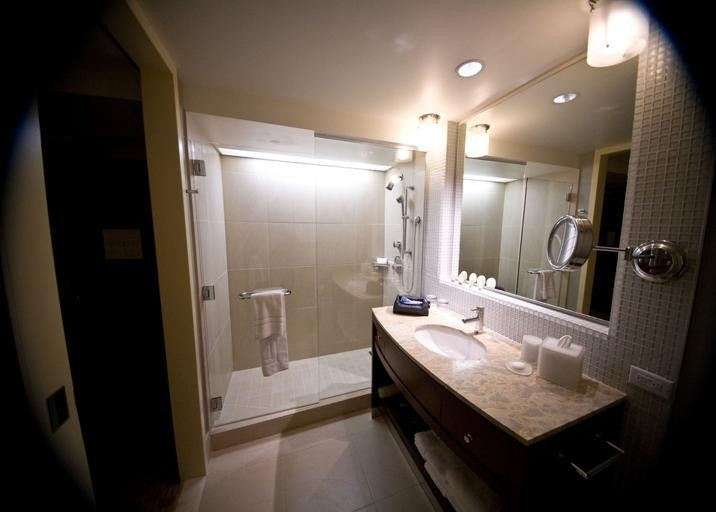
left=370, top=300, right=628, bottom=512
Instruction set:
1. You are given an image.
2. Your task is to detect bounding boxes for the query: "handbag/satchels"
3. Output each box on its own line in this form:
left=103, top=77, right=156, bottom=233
left=393, top=295, right=430, bottom=316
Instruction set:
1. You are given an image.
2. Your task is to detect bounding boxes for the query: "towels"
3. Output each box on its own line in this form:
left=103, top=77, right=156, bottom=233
left=251, top=290, right=290, bottom=377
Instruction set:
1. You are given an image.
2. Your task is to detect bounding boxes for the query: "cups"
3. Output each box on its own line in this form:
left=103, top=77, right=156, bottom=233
left=521, top=334, right=542, bottom=369
left=437, top=299, right=450, bottom=315
left=425, top=295, right=438, bottom=312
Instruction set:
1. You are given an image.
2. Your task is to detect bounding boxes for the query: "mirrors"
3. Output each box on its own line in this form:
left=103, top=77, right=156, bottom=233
left=448, top=45, right=639, bottom=333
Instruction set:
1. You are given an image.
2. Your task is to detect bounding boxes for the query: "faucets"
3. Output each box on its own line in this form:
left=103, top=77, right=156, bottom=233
left=461, top=306, right=485, bottom=335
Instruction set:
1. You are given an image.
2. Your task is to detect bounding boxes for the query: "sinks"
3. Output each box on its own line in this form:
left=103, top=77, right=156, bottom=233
left=414, top=324, right=488, bottom=360
